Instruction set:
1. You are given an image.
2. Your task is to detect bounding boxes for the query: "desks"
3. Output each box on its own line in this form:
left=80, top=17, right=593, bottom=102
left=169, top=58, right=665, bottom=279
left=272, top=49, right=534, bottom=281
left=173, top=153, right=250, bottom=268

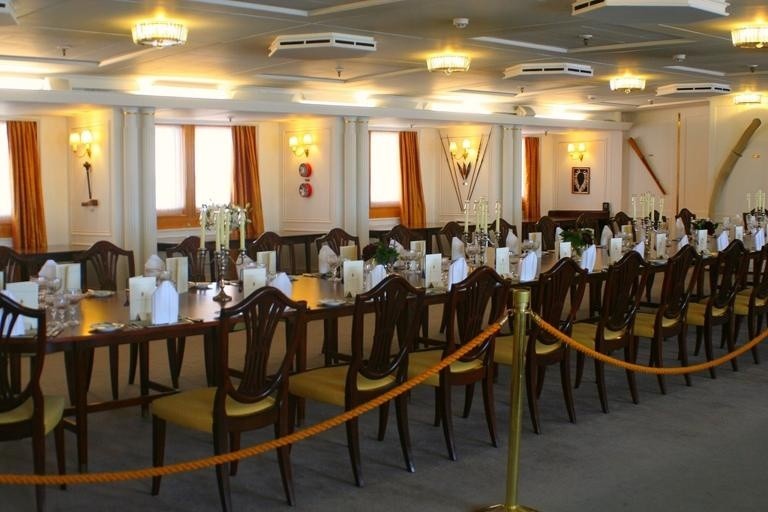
left=0, top=216, right=768, bottom=475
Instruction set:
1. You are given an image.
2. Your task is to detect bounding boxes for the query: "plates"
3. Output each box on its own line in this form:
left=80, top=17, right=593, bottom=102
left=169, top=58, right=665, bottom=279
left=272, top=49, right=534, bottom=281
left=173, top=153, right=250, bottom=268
left=320, top=298, right=347, bottom=306
left=649, top=259, right=668, bottom=264
left=93, top=289, right=115, bottom=297
left=89, top=321, right=124, bottom=333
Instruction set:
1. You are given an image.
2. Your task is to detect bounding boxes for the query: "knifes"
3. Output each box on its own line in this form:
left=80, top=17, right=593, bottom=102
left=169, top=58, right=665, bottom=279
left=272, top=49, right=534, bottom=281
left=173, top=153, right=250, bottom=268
left=46, top=321, right=67, bottom=336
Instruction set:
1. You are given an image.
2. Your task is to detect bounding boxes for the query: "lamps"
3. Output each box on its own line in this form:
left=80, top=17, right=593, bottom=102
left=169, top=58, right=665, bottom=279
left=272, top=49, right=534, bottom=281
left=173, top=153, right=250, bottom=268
left=610, top=78, right=645, bottom=94
left=70, top=130, right=92, bottom=159
left=448, top=140, right=470, bottom=161
left=426, top=54, right=470, bottom=75
left=731, top=27, right=768, bottom=49
left=289, top=133, right=311, bottom=158
left=131, top=23, right=189, bottom=50
left=568, top=143, right=586, bottom=162
left=734, top=93, right=762, bottom=105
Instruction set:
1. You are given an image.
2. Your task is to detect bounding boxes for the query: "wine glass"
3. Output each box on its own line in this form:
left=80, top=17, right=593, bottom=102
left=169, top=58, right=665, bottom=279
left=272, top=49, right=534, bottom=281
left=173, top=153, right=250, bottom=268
left=30, top=276, right=81, bottom=323
left=399, top=249, right=422, bottom=273
left=325, top=255, right=341, bottom=281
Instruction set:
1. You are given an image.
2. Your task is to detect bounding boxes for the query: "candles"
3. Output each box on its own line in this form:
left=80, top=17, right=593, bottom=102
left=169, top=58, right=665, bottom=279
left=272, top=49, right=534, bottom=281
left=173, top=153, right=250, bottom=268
left=631, top=191, right=665, bottom=223
left=745, top=189, right=766, bottom=214
left=198, top=201, right=252, bottom=249
left=462, top=196, right=501, bottom=234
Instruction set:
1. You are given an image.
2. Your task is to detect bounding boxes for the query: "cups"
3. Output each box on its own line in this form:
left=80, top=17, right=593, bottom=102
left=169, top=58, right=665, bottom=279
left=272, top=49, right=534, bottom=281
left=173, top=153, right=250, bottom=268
left=364, top=263, right=375, bottom=302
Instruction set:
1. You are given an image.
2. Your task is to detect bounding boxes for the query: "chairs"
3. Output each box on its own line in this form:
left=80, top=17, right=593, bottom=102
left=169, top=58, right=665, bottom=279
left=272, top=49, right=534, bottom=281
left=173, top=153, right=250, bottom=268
left=0, top=290, right=67, bottom=510
left=287, top=272, right=427, bottom=487
left=656, top=237, right=768, bottom=378
left=624, top=243, right=704, bottom=395
left=411, top=265, right=511, bottom=460
left=480, top=256, right=589, bottom=435
left=0, top=207, right=699, bottom=398
left=146, top=283, right=309, bottom=510
left=559, top=251, right=653, bottom=411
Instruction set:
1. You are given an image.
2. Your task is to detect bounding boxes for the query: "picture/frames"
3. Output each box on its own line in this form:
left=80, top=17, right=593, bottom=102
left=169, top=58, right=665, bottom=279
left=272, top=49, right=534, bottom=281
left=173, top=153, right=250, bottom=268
left=570, top=166, right=591, bottom=195
